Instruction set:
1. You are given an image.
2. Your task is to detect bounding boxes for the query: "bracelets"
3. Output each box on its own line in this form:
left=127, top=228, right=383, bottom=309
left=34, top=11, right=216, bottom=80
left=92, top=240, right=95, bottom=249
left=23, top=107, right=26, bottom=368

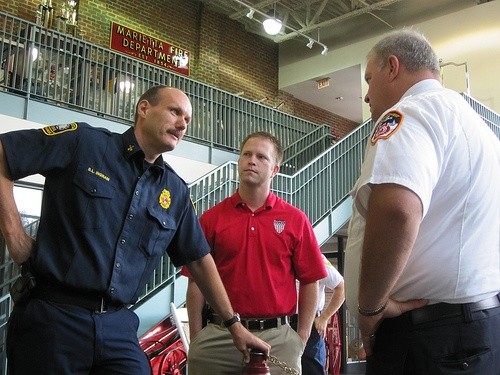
left=357, top=297, right=389, bottom=315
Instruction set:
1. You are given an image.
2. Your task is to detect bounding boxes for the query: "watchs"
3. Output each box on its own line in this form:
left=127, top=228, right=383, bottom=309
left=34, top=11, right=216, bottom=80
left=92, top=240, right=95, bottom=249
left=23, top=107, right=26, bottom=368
left=223, top=312, right=241, bottom=327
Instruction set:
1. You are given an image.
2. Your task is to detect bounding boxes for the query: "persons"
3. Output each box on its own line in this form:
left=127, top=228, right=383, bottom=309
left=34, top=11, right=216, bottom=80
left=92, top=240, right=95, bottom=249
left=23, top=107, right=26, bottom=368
left=291, top=254, right=345, bottom=375
left=0, top=87, right=271, bottom=375
left=344, top=28, right=500, bottom=375
left=181, top=133, right=328, bottom=375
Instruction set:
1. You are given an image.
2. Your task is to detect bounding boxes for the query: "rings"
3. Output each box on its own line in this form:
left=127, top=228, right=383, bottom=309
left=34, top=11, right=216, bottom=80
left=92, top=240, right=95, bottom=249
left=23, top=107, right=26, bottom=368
left=371, top=334, right=375, bottom=337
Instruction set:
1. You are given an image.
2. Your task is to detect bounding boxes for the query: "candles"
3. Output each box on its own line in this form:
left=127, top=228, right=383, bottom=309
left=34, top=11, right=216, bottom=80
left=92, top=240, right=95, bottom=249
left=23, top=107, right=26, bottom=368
left=36, top=0, right=78, bottom=37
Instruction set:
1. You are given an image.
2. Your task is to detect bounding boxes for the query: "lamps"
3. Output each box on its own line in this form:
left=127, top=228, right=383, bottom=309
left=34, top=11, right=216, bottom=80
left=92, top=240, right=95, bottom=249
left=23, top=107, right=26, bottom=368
left=120, top=72, right=135, bottom=93
left=29, top=46, right=39, bottom=62
left=234, top=0, right=328, bottom=56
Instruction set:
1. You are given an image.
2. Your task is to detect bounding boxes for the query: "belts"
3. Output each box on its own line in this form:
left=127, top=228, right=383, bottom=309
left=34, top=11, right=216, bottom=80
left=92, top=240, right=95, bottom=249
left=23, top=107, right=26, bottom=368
left=210, top=315, right=285, bottom=330
left=30, top=279, right=123, bottom=313
